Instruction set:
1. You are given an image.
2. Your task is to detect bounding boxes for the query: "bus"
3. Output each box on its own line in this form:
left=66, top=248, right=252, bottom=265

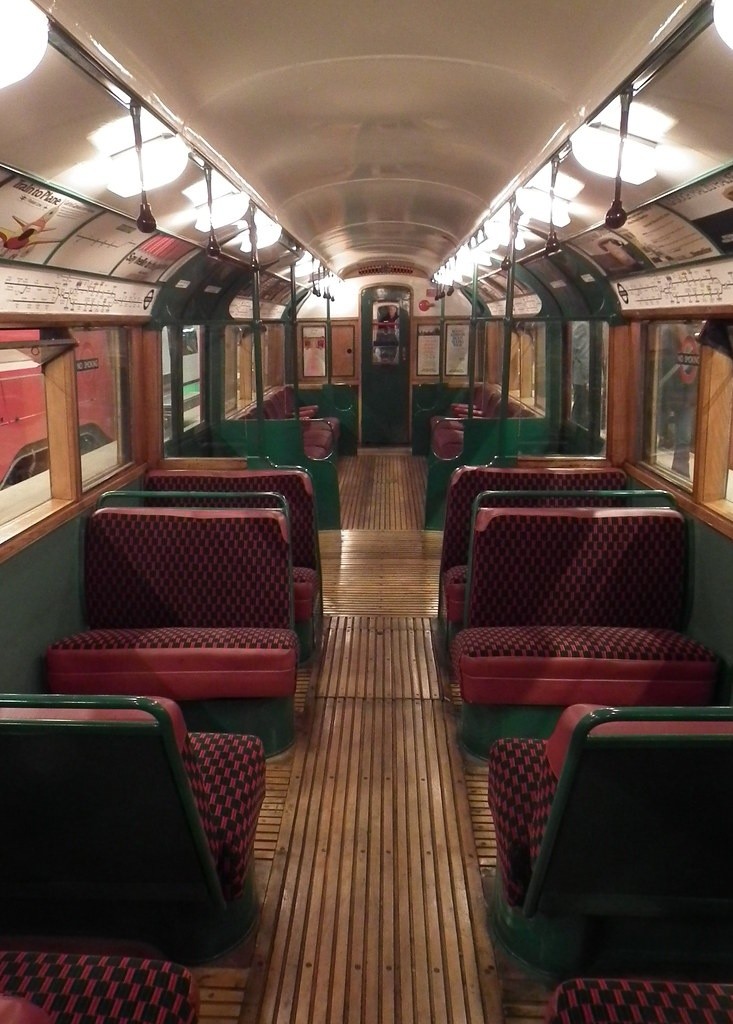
left=1, top=324, right=119, bottom=492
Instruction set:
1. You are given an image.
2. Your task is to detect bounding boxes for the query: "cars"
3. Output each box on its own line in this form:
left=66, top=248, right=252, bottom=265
left=161, top=326, right=200, bottom=400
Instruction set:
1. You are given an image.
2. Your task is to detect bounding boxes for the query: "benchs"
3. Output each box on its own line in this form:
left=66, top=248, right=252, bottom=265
left=0, top=457, right=324, bottom=1024
left=437, top=454, right=733, bottom=1023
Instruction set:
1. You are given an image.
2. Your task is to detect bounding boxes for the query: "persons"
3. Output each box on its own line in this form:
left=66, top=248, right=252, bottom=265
left=374, top=306, right=399, bottom=363
left=572, top=321, right=589, bottom=425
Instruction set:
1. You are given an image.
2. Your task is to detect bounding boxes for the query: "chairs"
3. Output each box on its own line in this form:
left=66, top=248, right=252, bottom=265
left=431, top=383, right=536, bottom=459
left=228, top=383, right=341, bottom=459
